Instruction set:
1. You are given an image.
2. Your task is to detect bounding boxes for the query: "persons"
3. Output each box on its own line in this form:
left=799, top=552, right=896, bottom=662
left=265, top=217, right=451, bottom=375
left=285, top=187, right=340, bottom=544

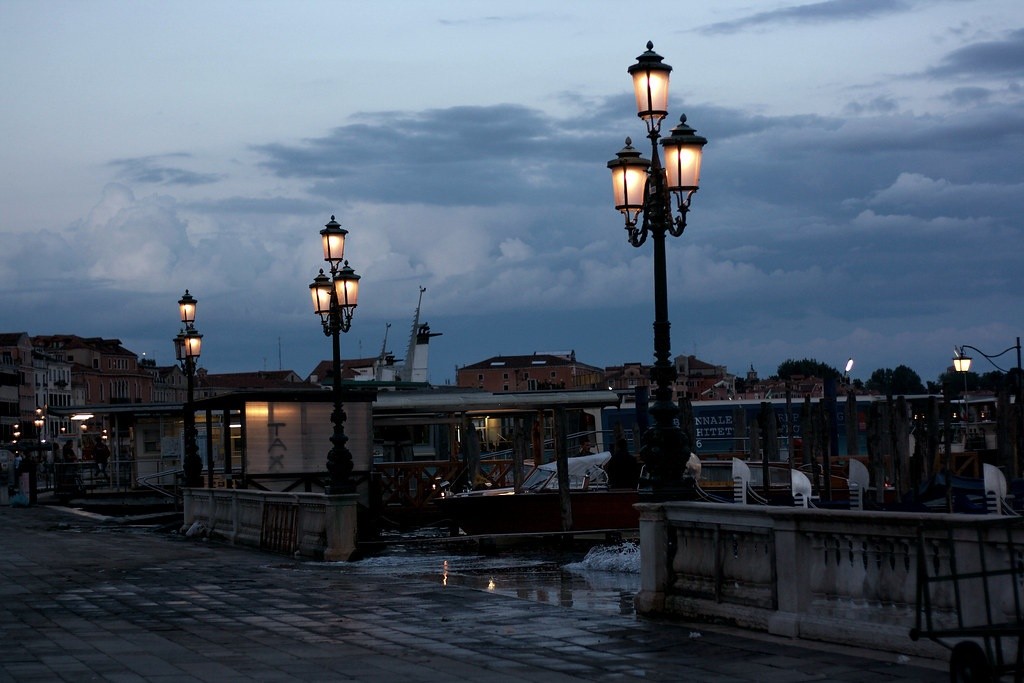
left=573, top=438, right=594, bottom=457
left=53, top=443, right=62, bottom=468
left=605, top=440, right=638, bottom=489
left=63, top=440, right=82, bottom=482
left=92, top=436, right=110, bottom=477
left=18, top=454, right=31, bottom=476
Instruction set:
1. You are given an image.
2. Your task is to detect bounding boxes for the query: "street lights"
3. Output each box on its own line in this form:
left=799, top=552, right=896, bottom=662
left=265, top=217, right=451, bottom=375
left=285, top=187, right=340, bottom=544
left=308, top=216, right=362, bottom=496
left=34, top=406, right=45, bottom=461
left=606, top=40, right=710, bottom=501
left=172, top=289, right=204, bottom=486
left=953, top=335, right=1024, bottom=478
